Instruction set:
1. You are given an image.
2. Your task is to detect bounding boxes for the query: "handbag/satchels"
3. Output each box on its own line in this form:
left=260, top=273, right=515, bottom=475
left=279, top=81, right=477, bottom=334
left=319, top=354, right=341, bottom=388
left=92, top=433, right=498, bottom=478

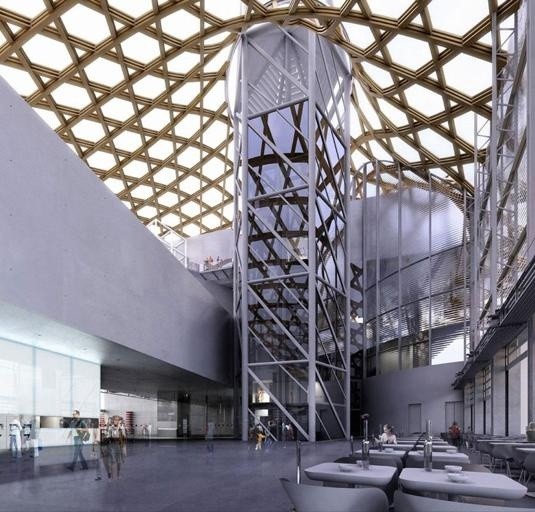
left=81, top=431, right=90, bottom=441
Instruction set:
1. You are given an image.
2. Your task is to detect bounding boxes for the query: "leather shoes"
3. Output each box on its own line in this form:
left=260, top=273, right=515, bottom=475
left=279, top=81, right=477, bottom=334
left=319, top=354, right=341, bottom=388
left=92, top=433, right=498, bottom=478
left=95, top=477, right=102, bottom=480
left=108, top=473, right=111, bottom=478
left=67, top=467, right=74, bottom=471
left=80, top=468, right=88, bottom=470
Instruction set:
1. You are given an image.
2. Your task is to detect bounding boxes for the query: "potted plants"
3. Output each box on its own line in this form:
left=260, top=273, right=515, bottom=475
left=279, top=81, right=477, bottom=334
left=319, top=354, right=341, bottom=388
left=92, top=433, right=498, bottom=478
left=361, top=414, right=369, bottom=441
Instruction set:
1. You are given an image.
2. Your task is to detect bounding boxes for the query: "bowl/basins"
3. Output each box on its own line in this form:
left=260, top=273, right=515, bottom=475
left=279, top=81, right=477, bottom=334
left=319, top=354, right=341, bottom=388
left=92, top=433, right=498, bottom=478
left=339, top=464, right=353, bottom=472
left=446, top=450, right=456, bottom=454
left=448, top=473, right=466, bottom=483
left=385, top=447, right=393, bottom=453
left=444, top=465, right=463, bottom=473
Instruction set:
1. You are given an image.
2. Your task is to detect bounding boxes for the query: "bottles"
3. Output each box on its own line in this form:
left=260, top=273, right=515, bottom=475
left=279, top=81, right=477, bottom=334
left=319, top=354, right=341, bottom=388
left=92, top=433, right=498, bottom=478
left=424, top=419, right=433, bottom=471
left=362, top=420, right=371, bottom=469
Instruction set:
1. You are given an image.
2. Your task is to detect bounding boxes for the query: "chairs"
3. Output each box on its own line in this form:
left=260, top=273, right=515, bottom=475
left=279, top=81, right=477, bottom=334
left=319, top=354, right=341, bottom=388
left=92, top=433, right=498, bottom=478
left=281, top=477, right=389, bottom=511
left=393, top=492, right=534, bottom=511
